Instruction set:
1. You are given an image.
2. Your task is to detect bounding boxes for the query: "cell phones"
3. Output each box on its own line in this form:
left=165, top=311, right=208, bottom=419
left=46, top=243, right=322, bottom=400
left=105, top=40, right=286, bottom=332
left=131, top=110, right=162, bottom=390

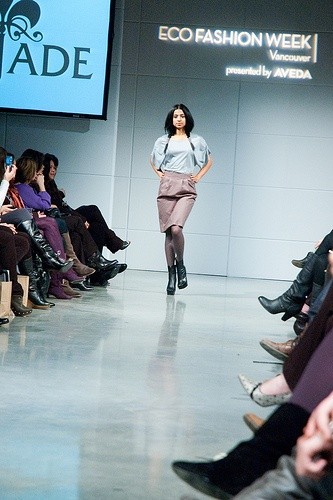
left=5, top=153, right=13, bottom=168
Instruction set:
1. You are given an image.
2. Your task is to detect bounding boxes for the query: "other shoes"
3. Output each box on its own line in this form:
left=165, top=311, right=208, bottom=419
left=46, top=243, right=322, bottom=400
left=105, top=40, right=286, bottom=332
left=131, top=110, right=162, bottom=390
left=260, top=338, right=300, bottom=361
left=107, top=264, right=127, bottom=273
left=119, top=241, right=131, bottom=250
left=291, top=252, right=313, bottom=268
left=166, top=264, right=177, bottom=295
left=90, top=268, right=119, bottom=286
left=176, top=261, right=188, bottom=289
left=10, top=295, right=32, bottom=316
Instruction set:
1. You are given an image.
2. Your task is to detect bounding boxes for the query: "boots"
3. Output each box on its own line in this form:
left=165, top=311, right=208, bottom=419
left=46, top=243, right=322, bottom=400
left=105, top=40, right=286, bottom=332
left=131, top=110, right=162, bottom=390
left=18, top=257, right=55, bottom=309
left=60, top=269, right=86, bottom=286
left=16, top=219, right=73, bottom=279
left=258, top=254, right=320, bottom=322
left=62, top=231, right=95, bottom=276
left=48, top=271, right=72, bottom=300
left=169, top=403, right=312, bottom=500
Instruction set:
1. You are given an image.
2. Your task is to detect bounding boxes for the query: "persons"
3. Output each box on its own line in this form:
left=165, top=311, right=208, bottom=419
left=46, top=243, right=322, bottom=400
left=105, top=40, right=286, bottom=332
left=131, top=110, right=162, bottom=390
left=150, top=104, right=212, bottom=294
left=0, top=146, right=131, bottom=325
left=171, top=229, right=333, bottom=500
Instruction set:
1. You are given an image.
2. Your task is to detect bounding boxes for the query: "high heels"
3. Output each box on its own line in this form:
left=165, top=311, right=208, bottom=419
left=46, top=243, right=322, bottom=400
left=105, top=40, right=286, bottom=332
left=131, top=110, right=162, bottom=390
left=237, top=373, right=291, bottom=407
left=60, top=280, right=82, bottom=297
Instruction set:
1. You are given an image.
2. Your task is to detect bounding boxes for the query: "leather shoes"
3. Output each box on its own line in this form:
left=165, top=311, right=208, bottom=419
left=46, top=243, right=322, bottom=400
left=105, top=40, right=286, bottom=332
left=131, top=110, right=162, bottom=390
left=87, top=254, right=118, bottom=268
